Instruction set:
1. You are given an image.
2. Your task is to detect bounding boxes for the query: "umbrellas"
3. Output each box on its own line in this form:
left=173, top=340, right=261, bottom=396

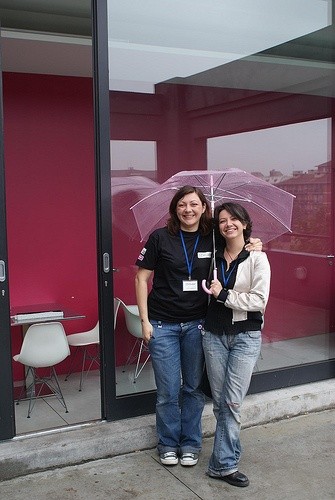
left=130, top=168, right=296, bottom=294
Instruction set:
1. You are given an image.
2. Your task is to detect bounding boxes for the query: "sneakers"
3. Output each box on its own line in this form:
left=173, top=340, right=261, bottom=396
left=180, top=453, right=199, bottom=466
left=159, top=451, right=178, bottom=464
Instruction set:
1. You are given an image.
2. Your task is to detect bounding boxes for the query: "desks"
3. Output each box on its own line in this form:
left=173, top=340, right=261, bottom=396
left=10, top=311, right=86, bottom=403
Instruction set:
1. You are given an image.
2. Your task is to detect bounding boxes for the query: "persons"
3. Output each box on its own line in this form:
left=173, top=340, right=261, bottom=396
left=135, top=185, right=263, bottom=465
left=202, top=202, right=270, bottom=486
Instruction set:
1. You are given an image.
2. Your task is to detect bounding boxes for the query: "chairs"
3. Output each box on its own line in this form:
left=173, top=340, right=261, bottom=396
left=116, top=297, right=152, bottom=384
left=12, top=322, right=71, bottom=419
left=64, top=298, right=120, bottom=392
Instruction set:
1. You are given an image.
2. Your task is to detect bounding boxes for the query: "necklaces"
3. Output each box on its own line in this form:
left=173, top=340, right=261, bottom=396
left=226, top=250, right=238, bottom=260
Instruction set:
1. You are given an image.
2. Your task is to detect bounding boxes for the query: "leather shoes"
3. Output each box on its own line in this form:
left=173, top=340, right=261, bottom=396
left=209, top=471, right=249, bottom=487
